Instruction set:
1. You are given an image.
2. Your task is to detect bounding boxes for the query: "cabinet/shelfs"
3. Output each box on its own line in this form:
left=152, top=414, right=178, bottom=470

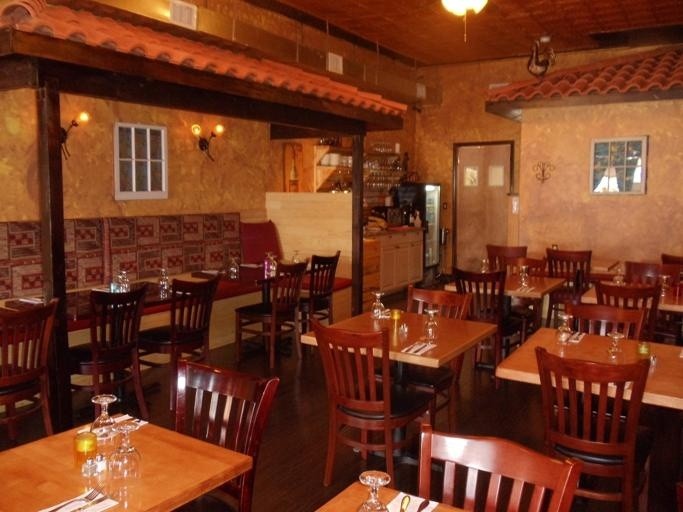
left=379, top=232, right=422, bottom=293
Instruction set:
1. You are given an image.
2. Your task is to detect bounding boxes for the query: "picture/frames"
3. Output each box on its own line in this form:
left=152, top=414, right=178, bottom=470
left=589, top=136, right=647, bottom=196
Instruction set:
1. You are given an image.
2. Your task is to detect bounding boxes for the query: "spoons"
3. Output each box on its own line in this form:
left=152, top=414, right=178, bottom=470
left=414, top=337, right=436, bottom=354
left=573, top=331, right=585, bottom=341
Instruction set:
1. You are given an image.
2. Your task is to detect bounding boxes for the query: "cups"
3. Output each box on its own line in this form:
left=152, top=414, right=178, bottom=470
left=89, top=393, right=117, bottom=439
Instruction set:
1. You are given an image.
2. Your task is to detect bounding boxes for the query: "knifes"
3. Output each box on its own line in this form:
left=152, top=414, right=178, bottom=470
left=70, top=494, right=114, bottom=512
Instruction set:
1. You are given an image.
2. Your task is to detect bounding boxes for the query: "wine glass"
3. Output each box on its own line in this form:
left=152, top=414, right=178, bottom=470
left=270, top=256, right=278, bottom=271
left=110, top=421, right=140, bottom=477
left=555, top=311, right=572, bottom=342
left=423, top=308, right=439, bottom=340
left=658, top=275, right=671, bottom=297
left=227, top=256, right=239, bottom=280
left=120, top=270, right=132, bottom=294
left=159, top=267, right=171, bottom=292
left=371, top=290, right=384, bottom=318
left=293, top=250, right=303, bottom=266
left=478, top=255, right=490, bottom=274
left=518, top=265, right=529, bottom=287
left=613, top=267, right=624, bottom=286
left=357, top=470, right=390, bottom=511
left=606, top=332, right=625, bottom=364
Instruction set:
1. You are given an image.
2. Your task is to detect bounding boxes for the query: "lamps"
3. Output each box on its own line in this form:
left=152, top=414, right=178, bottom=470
left=192, top=124, right=226, bottom=154
left=60, top=112, right=91, bottom=157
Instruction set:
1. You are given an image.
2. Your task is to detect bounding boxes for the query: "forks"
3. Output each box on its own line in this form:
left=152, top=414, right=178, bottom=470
left=49, top=482, right=107, bottom=512
left=405, top=334, right=427, bottom=356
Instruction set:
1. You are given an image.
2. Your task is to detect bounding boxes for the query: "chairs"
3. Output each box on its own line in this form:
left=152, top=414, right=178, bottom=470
left=552, top=301, right=643, bottom=423
left=416, top=423, right=581, bottom=512
left=547, top=246, right=592, bottom=325
left=533, top=346, right=653, bottom=512
left=69, top=288, right=146, bottom=418
left=450, top=266, right=531, bottom=386
left=510, top=257, right=545, bottom=335
left=309, top=318, right=436, bottom=487
left=484, top=242, right=523, bottom=274
left=139, top=273, right=218, bottom=412
left=172, top=360, right=278, bottom=512
left=375, top=284, right=473, bottom=436
left=593, top=278, right=654, bottom=339
left=300, top=250, right=340, bottom=330
left=627, top=261, right=680, bottom=293
left=0, top=299, right=60, bottom=438
left=235, top=259, right=309, bottom=368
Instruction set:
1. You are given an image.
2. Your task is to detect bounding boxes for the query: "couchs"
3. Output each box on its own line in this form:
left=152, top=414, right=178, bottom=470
left=0, top=213, right=352, bottom=350
